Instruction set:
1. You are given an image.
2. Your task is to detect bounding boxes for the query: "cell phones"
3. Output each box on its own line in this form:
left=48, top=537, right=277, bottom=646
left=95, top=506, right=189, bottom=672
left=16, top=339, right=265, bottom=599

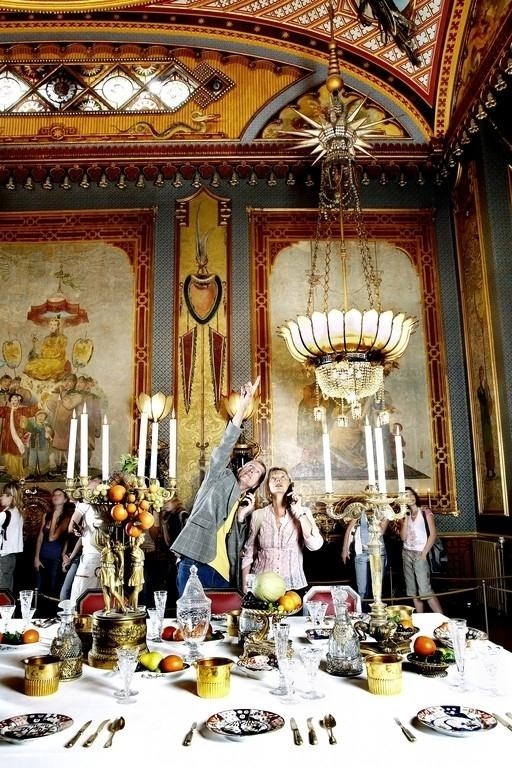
left=72, top=520, right=82, bottom=535
left=239, top=485, right=258, bottom=506
left=406, top=506, right=410, bottom=516
left=286, top=482, right=293, bottom=505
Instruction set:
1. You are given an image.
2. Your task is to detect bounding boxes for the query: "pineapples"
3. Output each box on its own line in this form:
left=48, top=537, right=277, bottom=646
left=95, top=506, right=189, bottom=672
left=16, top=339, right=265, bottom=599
left=108, top=454, right=140, bottom=484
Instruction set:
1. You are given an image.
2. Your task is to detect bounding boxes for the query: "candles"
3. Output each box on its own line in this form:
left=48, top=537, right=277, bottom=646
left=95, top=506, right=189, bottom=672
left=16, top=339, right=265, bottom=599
left=321, top=414, right=406, bottom=494
left=66, top=402, right=177, bottom=481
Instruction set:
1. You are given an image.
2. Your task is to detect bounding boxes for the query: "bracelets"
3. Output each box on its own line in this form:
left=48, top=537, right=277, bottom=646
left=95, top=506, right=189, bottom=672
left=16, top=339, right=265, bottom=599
left=298, top=513, right=306, bottom=518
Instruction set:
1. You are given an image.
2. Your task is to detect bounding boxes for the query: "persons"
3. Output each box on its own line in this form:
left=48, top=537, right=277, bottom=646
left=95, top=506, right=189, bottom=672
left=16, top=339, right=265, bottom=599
left=239, top=466, right=324, bottom=615
left=340, top=485, right=388, bottom=597
left=34, top=482, right=189, bottom=615
left=168, top=375, right=266, bottom=596
left=398, top=486, right=441, bottom=612
left=0, top=482, right=24, bottom=595
left=0, top=316, right=102, bottom=479
left=477, top=366, right=497, bottom=480
left=389, top=423, right=406, bottom=468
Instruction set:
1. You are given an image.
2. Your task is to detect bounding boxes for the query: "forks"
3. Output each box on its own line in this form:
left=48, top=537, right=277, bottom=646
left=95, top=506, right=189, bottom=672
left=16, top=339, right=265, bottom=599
left=38, top=615, right=57, bottom=630
left=182, top=720, right=200, bottom=748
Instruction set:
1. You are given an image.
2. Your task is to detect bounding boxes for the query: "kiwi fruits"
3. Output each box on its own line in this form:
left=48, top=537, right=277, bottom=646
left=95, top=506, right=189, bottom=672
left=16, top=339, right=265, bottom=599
left=162, top=655, right=185, bottom=671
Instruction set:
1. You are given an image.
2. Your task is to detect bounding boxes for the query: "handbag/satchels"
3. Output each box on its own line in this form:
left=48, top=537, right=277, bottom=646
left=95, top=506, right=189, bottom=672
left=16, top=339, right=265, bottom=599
left=427, top=537, right=450, bottom=574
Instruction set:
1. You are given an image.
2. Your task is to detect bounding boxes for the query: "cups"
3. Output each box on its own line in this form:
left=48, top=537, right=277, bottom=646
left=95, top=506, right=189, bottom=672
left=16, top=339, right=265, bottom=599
left=364, top=655, right=404, bottom=696
left=387, top=604, right=416, bottom=622
left=226, top=609, right=243, bottom=636
left=74, top=614, right=93, bottom=633
left=20, top=655, right=63, bottom=697
left=192, top=656, right=234, bottom=698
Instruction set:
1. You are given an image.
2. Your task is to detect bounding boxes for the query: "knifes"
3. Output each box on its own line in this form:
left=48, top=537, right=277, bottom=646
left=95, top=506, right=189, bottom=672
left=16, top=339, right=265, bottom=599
left=492, top=710, right=512, bottom=733
left=288, top=714, right=303, bottom=747
left=64, top=716, right=94, bottom=748
left=305, top=713, right=319, bottom=747
left=394, top=715, right=413, bottom=741
left=81, top=715, right=112, bottom=748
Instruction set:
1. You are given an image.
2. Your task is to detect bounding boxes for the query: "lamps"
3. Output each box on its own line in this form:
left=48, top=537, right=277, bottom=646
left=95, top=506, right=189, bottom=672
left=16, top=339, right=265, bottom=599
left=277, top=1, right=420, bottom=427
left=221, top=390, right=261, bottom=469
left=134, top=394, right=173, bottom=488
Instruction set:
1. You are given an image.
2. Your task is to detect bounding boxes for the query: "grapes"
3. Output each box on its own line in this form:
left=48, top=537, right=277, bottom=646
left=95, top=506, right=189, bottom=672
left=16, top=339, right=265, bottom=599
left=149, top=492, right=164, bottom=513
left=241, top=591, right=278, bottom=610
left=396, top=624, right=412, bottom=632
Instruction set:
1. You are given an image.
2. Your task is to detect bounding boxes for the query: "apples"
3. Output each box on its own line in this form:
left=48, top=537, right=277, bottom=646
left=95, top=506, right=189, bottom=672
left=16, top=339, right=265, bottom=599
left=161, top=623, right=213, bottom=641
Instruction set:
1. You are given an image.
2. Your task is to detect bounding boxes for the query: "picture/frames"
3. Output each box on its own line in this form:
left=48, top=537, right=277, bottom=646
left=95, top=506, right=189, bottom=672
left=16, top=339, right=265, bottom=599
left=0, top=207, right=156, bottom=492
left=248, top=209, right=460, bottom=512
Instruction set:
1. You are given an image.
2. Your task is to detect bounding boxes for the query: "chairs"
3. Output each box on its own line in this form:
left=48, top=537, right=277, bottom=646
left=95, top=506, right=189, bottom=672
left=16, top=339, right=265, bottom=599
left=204, top=592, right=242, bottom=614
left=304, top=585, right=363, bottom=614
left=76, top=589, right=105, bottom=615
left=0, top=587, right=16, bottom=619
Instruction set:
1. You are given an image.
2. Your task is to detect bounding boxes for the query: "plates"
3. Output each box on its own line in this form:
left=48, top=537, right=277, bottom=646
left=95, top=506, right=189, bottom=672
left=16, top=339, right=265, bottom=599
left=0, top=712, right=74, bottom=744
left=432, top=624, right=488, bottom=648
left=163, top=637, right=222, bottom=649
left=141, top=663, right=189, bottom=680
left=0, top=641, right=41, bottom=656
left=333, top=611, right=368, bottom=623
left=301, top=627, right=366, bottom=643
left=205, top=708, right=286, bottom=742
left=207, top=611, right=229, bottom=625
left=413, top=704, right=496, bottom=736
left=407, top=652, right=449, bottom=680
left=237, top=655, right=279, bottom=682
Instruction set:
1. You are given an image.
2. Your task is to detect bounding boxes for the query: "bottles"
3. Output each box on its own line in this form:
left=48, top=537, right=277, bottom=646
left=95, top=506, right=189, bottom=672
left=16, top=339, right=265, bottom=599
left=54, top=599, right=84, bottom=681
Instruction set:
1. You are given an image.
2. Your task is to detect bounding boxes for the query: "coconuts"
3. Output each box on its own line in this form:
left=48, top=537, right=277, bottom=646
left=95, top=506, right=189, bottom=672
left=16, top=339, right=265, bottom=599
left=252, top=571, right=287, bottom=603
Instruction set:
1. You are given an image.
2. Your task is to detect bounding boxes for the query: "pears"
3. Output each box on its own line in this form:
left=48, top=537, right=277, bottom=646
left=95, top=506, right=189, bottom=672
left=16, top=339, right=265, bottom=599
left=136, top=651, right=162, bottom=673
left=278, top=589, right=302, bottom=612
left=397, top=620, right=413, bottom=629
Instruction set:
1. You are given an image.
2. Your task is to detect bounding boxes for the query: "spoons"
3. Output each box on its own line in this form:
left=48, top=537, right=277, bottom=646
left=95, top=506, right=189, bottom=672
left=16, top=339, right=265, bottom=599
left=323, top=710, right=339, bottom=745
left=102, top=714, right=128, bottom=750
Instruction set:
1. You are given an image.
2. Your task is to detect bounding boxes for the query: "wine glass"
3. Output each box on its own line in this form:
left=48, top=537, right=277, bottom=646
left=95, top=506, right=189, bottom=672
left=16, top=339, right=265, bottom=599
left=269, top=620, right=324, bottom=701
left=109, top=644, right=141, bottom=704
left=305, top=597, right=329, bottom=628
left=1, top=587, right=39, bottom=632
left=136, top=586, right=166, bottom=643
left=445, top=617, right=470, bottom=689
